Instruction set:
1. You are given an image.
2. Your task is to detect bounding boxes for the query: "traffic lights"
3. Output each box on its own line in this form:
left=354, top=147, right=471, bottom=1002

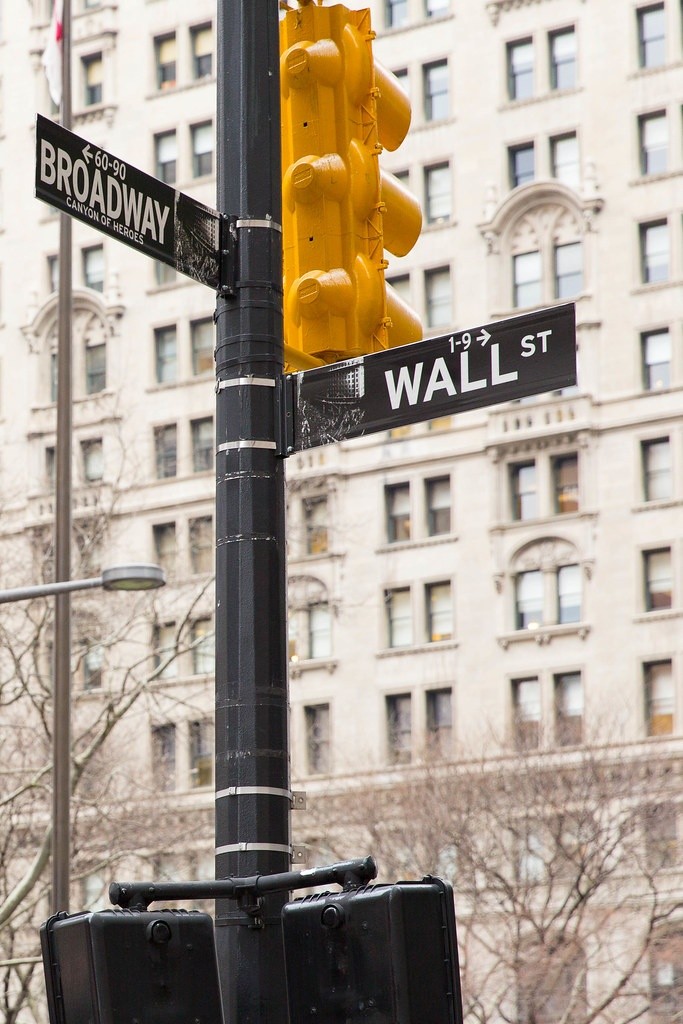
left=274, top=4, right=429, bottom=361
